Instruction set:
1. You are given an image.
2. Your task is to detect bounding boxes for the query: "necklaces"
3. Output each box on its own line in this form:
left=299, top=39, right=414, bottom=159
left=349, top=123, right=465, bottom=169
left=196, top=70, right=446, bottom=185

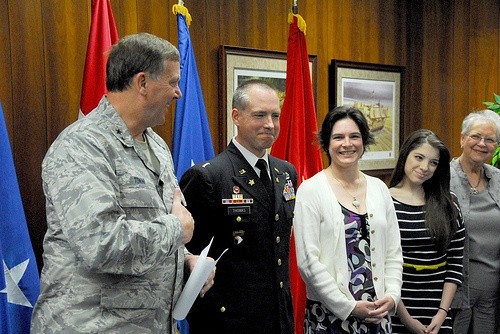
left=458, top=159, right=483, bottom=195
left=334, top=168, right=363, bottom=209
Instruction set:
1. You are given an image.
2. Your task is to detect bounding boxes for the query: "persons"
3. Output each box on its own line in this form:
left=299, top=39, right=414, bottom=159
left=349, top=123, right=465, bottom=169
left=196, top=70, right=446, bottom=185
left=450, top=109, right=500, bottom=334
left=293, top=105, right=401, bottom=334
left=387, top=129, right=465, bottom=334
left=32, top=32, right=215, bottom=334
left=179, top=79, right=299, bottom=334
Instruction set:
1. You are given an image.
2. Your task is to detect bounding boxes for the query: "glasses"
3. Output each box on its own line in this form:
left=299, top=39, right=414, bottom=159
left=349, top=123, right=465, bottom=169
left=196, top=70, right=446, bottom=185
left=465, top=133, right=497, bottom=144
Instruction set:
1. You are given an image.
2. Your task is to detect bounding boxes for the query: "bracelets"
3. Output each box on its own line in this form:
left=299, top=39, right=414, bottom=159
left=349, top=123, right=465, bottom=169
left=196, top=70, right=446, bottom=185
left=438, top=307, right=449, bottom=314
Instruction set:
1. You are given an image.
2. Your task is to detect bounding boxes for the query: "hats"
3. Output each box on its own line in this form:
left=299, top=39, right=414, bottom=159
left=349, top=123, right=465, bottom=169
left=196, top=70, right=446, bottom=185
left=255, top=159, right=275, bottom=211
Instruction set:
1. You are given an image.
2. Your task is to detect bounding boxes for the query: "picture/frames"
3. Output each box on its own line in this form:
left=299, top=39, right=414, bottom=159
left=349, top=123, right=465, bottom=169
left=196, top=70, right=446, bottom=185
left=219, top=45, right=317, bottom=155
left=331, top=58, right=406, bottom=176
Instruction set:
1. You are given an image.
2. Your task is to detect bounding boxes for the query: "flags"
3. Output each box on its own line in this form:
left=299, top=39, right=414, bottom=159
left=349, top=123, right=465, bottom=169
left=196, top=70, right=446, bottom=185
left=78, top=0, right=121, bottom=118
left=170, top=6, right=214, bottom=334
left=0, top=105, right=41, bottom=334
left=271, top=14, right=324, bottom=334
